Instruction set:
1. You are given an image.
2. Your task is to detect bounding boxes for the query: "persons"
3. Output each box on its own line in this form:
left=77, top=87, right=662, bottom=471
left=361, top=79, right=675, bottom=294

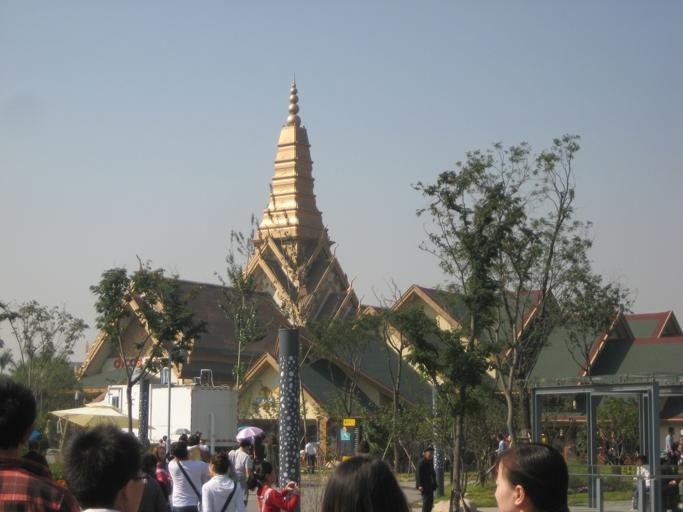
left=627, top=421, right=683, bottom=511
left=0, top=372, right=84, bottom=512
left=492, top=442, right=570, bottom=511
left=415, top=446, right=437, bottom=512
left=23, top=431, right=301, bottom=511
left=303, top=439, right=320, bottom=472
left=319, top=453, right=411, bottom=511
